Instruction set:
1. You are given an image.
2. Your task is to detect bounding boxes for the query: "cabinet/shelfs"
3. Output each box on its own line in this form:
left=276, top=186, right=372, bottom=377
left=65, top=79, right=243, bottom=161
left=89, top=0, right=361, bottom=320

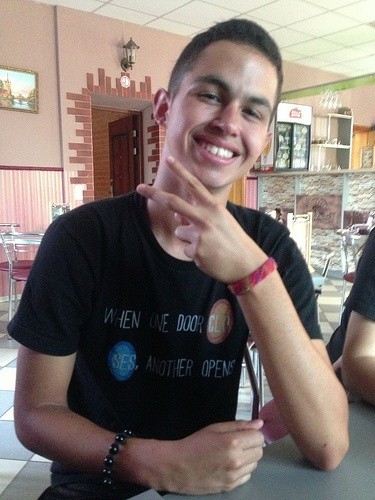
left=311, top=112, right=354, bottom=173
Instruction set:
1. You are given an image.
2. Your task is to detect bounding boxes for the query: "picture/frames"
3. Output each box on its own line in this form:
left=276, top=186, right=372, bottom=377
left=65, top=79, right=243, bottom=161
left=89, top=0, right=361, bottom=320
left=0, top=65, right=39, bottom=114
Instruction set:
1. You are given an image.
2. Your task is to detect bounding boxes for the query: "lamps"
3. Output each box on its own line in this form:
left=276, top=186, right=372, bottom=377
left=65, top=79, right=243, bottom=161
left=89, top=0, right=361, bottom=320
left=120, top=37, right=140, bottom=72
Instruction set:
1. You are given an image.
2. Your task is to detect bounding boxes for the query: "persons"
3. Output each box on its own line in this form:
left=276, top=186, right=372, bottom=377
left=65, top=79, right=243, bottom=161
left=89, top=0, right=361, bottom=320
left=325, top=227, right=375, bottom=408
left=7, top=18, right=348, bottom=500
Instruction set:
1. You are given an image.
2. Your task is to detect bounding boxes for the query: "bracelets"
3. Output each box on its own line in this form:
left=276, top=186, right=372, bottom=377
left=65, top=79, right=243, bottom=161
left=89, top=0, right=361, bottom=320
left=102, top=426, right=133, bottom=489
left=227, top=254, right=277, bottom=296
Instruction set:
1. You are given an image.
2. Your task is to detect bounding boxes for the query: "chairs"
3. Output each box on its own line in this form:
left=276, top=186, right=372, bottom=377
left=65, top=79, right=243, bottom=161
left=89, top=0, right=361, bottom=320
left=334, top=223, right=372, bottom=324
left=0, top=223, right=45, bottom=340
